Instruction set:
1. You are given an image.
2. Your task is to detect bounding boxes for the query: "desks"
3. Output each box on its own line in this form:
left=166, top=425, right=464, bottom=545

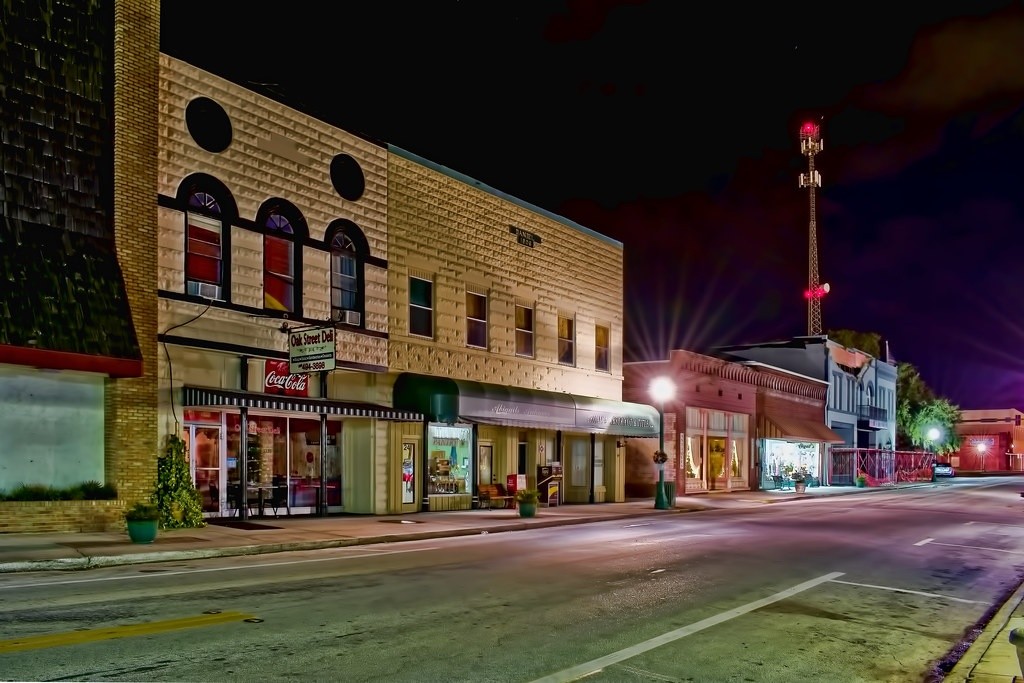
left=246, top=486, right=279, bottom=517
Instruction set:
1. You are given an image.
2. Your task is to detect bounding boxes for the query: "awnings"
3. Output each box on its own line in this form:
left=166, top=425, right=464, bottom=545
left=392, top=372, right=662, bottom=438
left=758, top=414, right=845, bottom=442
left=183, top=386, right=424, bottom=421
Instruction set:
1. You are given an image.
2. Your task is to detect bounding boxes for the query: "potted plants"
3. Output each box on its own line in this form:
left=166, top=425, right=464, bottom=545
left=795, top=479, right=807, bottom=494
left=856, top=476, right=864, bottom=487
left=124, top=502, right=162, bottom=544
left=515, top=489, right=541, bottom=517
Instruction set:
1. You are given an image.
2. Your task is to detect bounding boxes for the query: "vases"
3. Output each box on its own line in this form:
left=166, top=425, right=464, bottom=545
left=656, top=463, right=663, bottom=470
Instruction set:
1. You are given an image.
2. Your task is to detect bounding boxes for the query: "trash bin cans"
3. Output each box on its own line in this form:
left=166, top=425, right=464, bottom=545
left=657, top=482, right=675, bottom=507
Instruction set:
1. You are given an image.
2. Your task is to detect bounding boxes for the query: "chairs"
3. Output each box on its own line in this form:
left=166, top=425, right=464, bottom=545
left=209, top=482, right=291, bottom=517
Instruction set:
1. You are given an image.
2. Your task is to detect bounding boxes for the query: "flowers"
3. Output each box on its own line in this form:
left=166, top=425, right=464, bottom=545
left=652, top=450, right=667, bottom=464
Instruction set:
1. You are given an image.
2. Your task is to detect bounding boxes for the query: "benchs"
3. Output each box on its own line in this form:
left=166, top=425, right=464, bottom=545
left=477, top=483, right=516, bottom=511
left=772, top=476, right=790, bottom=490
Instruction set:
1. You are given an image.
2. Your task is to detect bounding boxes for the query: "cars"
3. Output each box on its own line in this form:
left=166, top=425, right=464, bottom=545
left=935, top=463, right=952, bottom=476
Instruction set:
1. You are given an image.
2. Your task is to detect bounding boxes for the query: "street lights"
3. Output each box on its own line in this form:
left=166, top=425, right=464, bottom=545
left=976, top=444, right=986, bottom=472
left=927, top=429, right=940, bottom=482
left=648, top=376, right=675, bottom=508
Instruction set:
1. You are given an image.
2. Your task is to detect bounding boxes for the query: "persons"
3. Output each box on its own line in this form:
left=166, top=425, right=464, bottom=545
left=434, top=456, right=440, bottom=476
left=209, top=471, right=218, bottom=490
